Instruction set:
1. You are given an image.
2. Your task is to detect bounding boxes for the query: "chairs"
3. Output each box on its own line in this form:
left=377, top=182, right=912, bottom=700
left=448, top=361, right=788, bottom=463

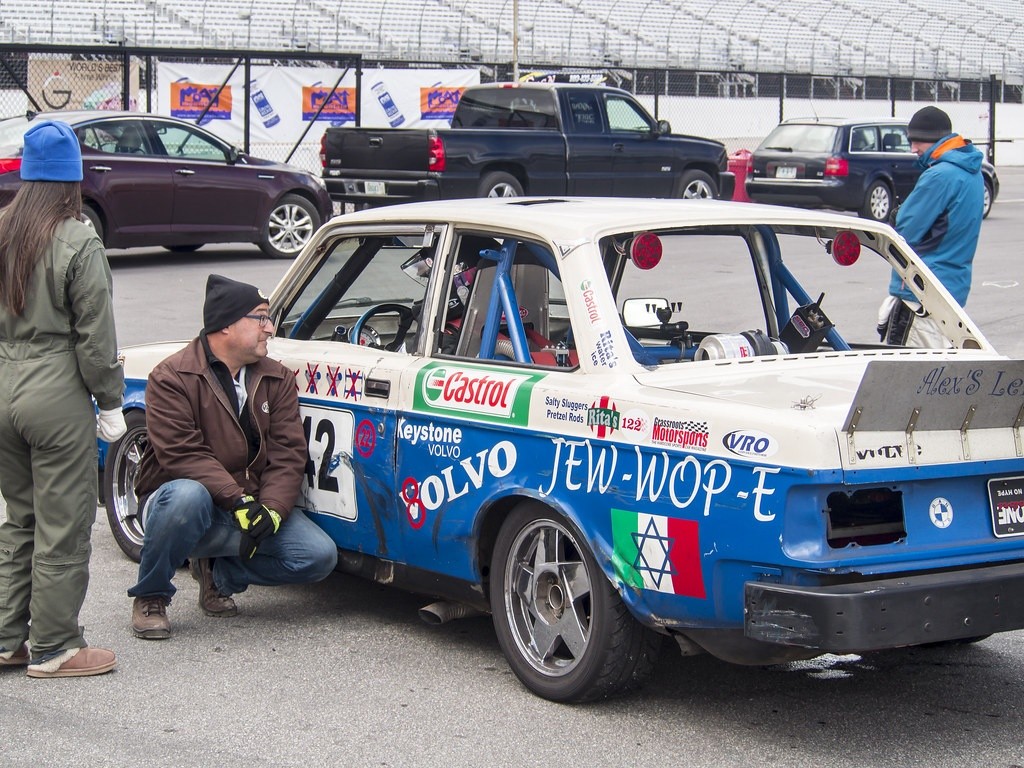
left=114, top=127, right=142, bottom=154
left=444, top=265, right=553, bottom=358
left=883, top=134, right=905, bottom=152
left=852, top=132, right=867, bottom=149
left=508, top=105, right=536, bottom=127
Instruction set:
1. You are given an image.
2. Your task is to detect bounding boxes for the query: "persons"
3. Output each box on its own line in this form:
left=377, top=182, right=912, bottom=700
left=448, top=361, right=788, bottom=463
left=0, top=120, right=127, bottom=679
left=127, top=274, right=337, bottom=639
left=879, top=106, right=985, bottom=349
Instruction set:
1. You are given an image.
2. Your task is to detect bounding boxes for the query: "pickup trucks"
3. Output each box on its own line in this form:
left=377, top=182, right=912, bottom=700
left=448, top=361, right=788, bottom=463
left=317, top=80, right=728, bottom=205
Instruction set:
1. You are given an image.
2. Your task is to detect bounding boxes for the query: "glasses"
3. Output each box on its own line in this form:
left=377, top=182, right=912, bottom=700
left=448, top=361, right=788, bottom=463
left=244, top=314, right=271, bottom=326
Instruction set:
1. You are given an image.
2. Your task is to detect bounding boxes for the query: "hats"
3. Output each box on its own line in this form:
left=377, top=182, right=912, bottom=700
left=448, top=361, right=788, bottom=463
left=203, top=274, right=270, bottom=335
left=907, top=107, right=952, bottom=143
left=20, top=121, right=83, bottom=182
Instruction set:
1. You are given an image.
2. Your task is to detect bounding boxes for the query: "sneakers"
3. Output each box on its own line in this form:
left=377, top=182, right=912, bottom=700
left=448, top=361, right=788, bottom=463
left=189, top=557, right=237, bottom=617
left=131, top=595, right=171, bottom=639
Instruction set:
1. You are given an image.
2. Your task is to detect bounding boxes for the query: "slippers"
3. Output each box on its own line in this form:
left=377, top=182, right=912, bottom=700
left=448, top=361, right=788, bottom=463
left=0, top=633, right=31, bottom=664
left=26, top=647, right=117, bottom=678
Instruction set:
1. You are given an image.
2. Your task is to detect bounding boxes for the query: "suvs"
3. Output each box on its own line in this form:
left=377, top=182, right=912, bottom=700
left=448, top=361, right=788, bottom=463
left=745, top=115, right=1000, bottom=226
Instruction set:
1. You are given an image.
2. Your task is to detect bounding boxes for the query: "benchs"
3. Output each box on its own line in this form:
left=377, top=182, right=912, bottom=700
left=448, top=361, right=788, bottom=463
left=0, top=0, right=1024, bottom=83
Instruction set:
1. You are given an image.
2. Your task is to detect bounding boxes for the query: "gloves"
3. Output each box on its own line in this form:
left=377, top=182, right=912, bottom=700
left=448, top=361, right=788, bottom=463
left=95, top=407, right=127, bottom=442
left=230, top=495, right=256, bottom=534
left=245, top=503, right=282, bottom=546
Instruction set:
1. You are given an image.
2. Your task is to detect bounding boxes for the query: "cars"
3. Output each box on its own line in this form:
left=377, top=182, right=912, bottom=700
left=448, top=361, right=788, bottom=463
left=89, top=195, right=1024, bottom=709
left=0, top=110, right=335, bottom=262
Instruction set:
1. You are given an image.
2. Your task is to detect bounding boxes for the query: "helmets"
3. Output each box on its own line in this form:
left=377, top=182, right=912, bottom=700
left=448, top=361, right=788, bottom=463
left=414, top=235, right=502, bottom=324
